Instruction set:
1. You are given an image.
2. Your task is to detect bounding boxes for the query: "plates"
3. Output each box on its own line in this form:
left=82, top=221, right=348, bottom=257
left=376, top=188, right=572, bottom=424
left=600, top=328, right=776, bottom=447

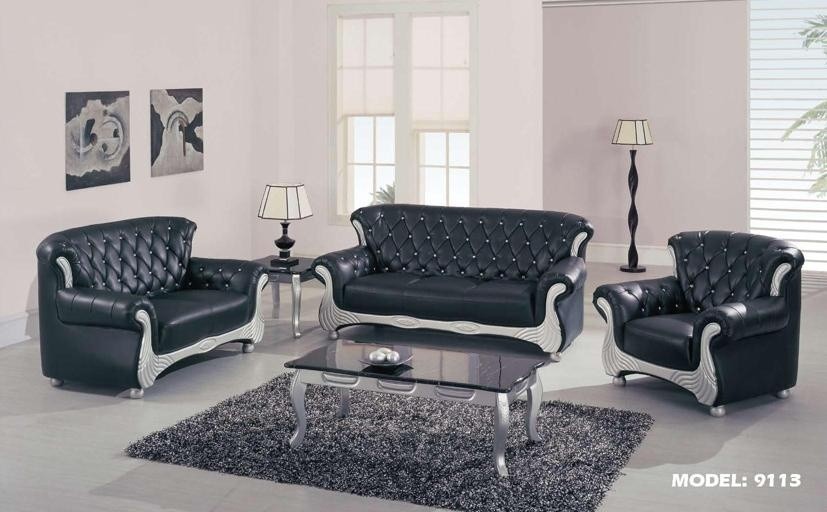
left=359, top=345, right=414, bottom=367
left=361, top=364, right=413, bottom=377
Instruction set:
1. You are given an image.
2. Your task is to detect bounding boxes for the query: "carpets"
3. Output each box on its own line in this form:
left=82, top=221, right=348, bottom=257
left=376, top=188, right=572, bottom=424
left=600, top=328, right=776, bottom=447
left=123, top=371, right=654, bottom=512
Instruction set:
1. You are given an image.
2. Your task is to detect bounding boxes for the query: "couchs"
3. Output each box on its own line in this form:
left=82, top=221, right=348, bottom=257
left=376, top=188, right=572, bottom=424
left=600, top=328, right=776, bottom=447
left=35, top=216, right=271, bottom=399
left=312, top=203, right=594, bottom=363
left=593, top=229, right=805, bottom=417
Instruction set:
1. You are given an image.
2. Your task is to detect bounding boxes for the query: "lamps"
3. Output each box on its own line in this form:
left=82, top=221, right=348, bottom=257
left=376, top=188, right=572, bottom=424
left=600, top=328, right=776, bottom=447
left=258, top=183, right=314, bottom=267
left=611, top=119, right=653, bottom=273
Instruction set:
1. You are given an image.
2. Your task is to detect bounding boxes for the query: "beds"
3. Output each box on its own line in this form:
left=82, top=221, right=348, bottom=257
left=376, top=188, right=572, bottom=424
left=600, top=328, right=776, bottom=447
left=251, top=255, right=317, bottom=337
